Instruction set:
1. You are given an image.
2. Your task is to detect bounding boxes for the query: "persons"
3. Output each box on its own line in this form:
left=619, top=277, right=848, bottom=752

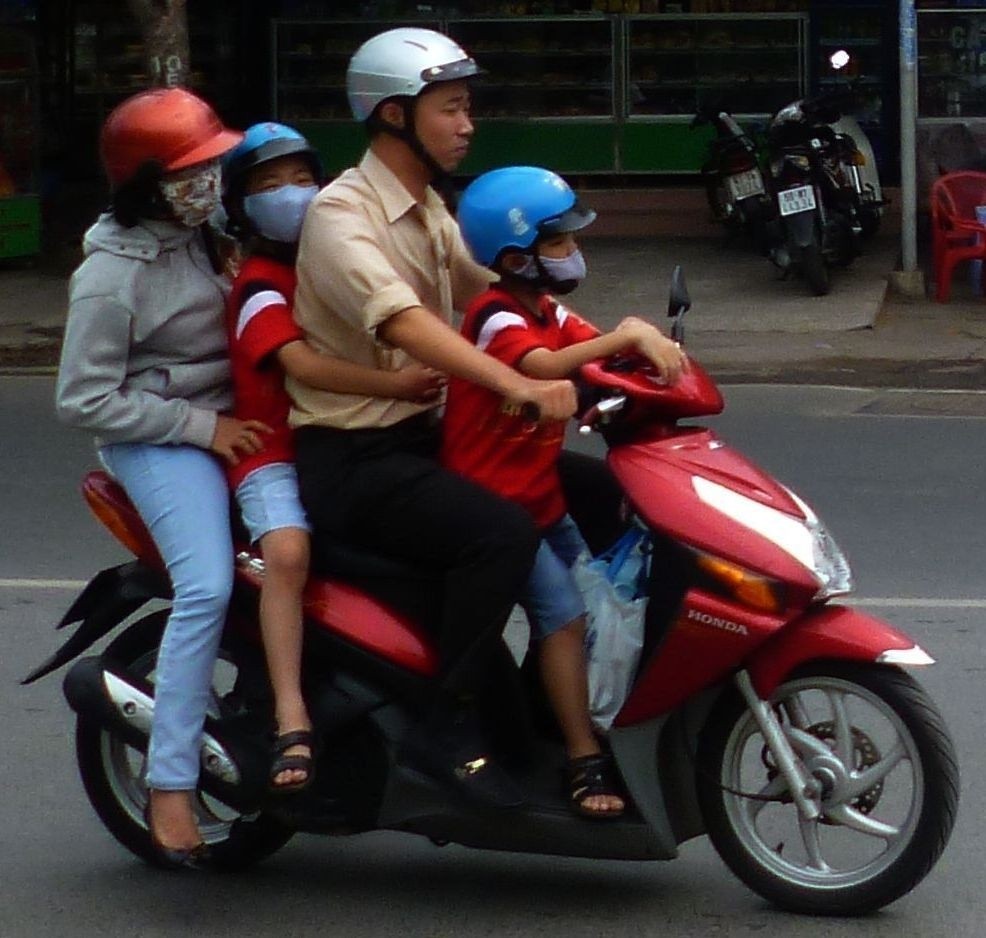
left=219, top=120, right=452, bottom=789
left=436, top=165, right=691, bottom=819
left=284, top=25, right=664, bottom=814
left=54, top=86, right=300, bottom=880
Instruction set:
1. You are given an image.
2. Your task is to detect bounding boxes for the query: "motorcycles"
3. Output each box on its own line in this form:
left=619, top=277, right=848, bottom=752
left=692, top=75, right=774, bottom=257
left=21, top=265, right=963, bottom=918
left=764, top=49, right=891, bottom=296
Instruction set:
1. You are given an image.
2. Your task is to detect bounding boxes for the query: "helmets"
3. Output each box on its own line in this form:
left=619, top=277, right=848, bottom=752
left=456, top=164, right=597, bottom=268
left=98, top=87, right=245, bottom=184
left=221, top=121, right=324, bottom=193
left=346, top=27, right=484, bottom=123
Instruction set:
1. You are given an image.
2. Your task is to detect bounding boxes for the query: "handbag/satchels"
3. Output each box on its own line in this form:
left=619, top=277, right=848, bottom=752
left=516, top=528, right=648, bottom=739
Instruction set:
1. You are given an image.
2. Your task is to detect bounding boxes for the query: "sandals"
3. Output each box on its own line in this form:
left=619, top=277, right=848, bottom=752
left=267, top=723, right=320, bottom=799
left=554, top=744, right=627, bottom=818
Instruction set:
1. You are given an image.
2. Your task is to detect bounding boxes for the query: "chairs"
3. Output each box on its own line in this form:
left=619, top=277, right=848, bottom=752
left=931, top=171, right=984, bottom=304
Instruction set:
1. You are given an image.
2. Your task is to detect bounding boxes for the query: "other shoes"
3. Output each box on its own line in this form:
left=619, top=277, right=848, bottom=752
left=444, top=743, right=528, bottom=813
left=148, top=787, right=207, bottom=872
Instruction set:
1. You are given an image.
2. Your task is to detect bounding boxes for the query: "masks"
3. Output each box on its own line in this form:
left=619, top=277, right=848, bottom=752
left=162, top=166, right=222, bottom=225
left=514, top=249, right=587, bottom=282
left=242, top=183, right=319, bottom=242
